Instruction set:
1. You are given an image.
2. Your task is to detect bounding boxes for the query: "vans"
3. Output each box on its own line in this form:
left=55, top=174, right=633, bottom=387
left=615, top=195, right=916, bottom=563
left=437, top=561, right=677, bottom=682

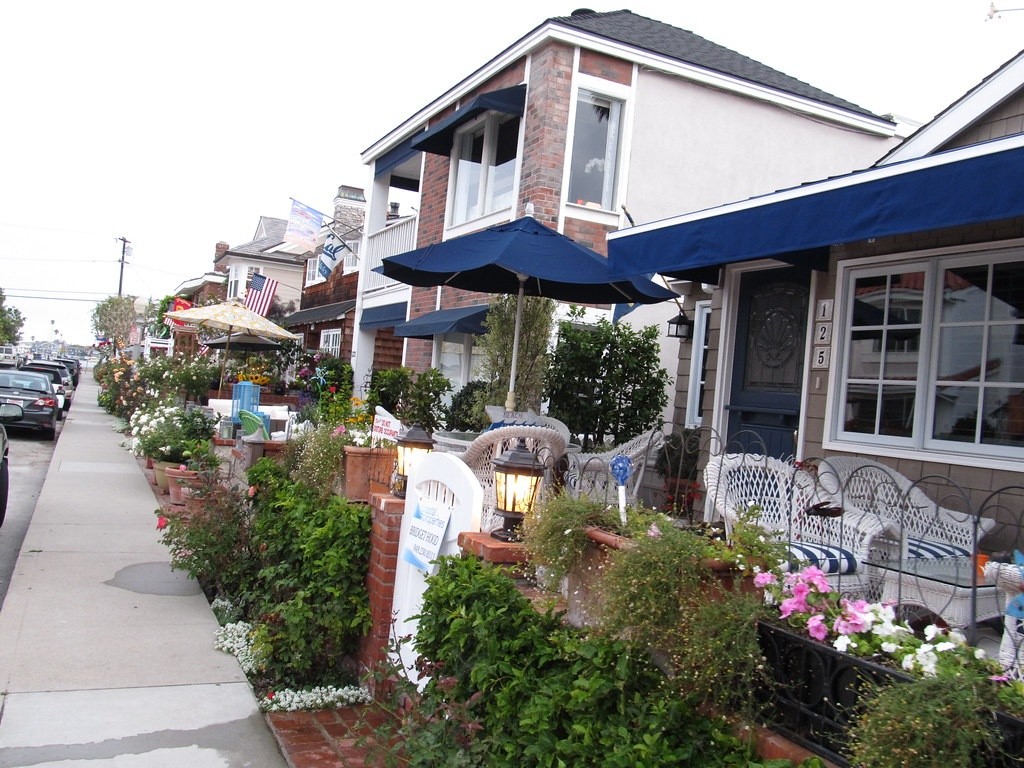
left=0, top=346, right=17, bottom=368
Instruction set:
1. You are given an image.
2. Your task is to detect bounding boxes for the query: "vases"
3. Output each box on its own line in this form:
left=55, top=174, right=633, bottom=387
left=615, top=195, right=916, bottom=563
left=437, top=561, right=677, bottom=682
left=184, top=494, right=223, bottom=519
left=340, top=443, right=398, bottom=504
left=153, top=460, right=185, bottom=494
left=165, top=464, right=205, bottom=505
left=146, top=453, right=156, bottom=470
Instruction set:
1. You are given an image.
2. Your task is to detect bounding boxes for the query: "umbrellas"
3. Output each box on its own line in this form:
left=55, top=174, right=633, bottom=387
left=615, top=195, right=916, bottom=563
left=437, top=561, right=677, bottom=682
left=160, top=300, right=300, bottom=399
left=381, top=201, right=681, bottom=416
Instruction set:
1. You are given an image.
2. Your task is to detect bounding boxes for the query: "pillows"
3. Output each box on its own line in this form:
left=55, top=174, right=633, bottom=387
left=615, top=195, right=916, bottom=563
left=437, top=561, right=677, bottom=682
left=906, top=537, right=972, bottom=560
left=769, top=539, right=858, bottom=577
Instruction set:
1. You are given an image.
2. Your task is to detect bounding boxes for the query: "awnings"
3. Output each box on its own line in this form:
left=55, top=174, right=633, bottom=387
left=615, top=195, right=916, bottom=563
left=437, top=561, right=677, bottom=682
left=391, top=305, right=503, bottom=340
left=410, top=81, right=527, bottom=152
left=361, top=299, right=407, bottom=331
left=600, top=133, right=1024, bottom=285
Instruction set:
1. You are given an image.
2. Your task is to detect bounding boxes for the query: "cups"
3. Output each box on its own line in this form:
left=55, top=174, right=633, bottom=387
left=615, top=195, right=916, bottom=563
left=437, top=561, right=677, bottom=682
left=977, top=553, right=991, bottom=580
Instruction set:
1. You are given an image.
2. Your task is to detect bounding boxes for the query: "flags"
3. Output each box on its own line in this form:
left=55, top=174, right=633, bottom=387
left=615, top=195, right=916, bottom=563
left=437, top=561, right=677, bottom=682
left=161, top=298, right=191, bottom=331
left=283, top=200, right=326, bottom=254
left=243, top=274, right=278, bottom=319
left=612, top=209, right=657, bottom=326
left=318, top=230, right=352, bottom=281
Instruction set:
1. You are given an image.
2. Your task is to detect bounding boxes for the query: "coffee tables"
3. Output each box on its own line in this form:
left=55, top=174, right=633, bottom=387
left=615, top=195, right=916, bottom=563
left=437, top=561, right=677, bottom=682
left=861, top=556, right=1007, bottom=637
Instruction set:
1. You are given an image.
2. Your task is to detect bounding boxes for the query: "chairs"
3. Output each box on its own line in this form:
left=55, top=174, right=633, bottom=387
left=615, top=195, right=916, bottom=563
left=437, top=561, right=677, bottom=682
left=447, top=425, right=568, bottom=535
left=702, top=452, right=906, bottom=600
left=817, top=455, right=997, bottom=559
left=569, top=428, right=665, bottom=512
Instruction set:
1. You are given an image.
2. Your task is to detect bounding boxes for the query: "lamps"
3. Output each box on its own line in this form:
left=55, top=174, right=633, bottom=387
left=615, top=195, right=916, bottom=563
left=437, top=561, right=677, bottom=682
left=393, top=419, right=437, bottom=499
left=487, top=436, right=550, bottom=544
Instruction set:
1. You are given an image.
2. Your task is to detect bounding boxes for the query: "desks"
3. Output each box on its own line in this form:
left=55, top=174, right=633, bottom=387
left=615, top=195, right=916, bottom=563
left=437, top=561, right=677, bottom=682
left=431, top=431, right=485, bottom=454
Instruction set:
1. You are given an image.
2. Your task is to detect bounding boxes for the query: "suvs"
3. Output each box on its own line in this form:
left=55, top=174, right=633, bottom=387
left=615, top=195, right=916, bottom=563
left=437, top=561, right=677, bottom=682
left=23, top=359, right=74, bottom=409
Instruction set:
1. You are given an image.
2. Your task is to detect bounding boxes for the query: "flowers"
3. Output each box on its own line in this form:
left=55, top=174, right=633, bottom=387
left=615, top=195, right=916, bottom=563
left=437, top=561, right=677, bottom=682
left=751, top=565, right=1022, bottom=722
left=129, top=401, right=217, bottom=471
left=331, top=396, right=398, bottom=448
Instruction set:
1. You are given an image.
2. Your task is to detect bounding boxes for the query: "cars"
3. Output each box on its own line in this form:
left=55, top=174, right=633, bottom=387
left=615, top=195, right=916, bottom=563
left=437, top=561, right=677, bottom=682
left=0, top=369, right=66, bottom=439
left=10, top=365, right=69, bottom=419
left=52, top=359, right=81, bottom=391
